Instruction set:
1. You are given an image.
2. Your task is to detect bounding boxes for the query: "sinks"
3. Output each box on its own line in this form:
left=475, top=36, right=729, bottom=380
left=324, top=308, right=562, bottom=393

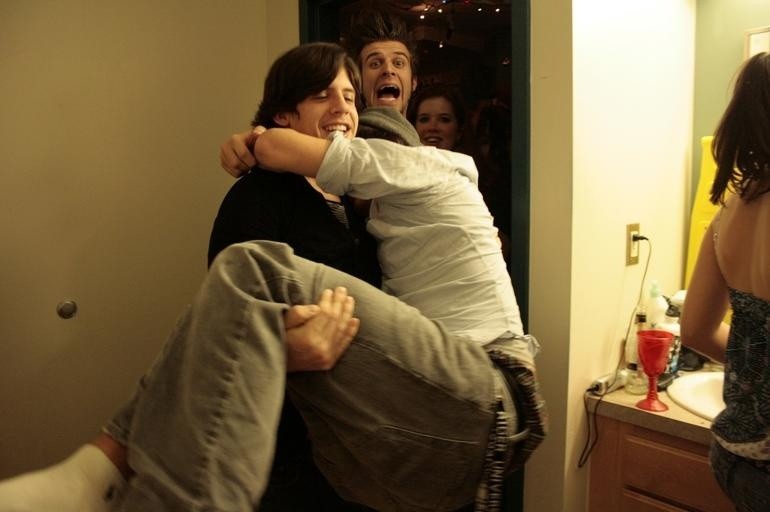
left=666, top=371, right=727, bottom=421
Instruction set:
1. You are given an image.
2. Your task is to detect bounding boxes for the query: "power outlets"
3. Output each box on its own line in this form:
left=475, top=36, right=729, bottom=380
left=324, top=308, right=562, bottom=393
left=627, top=224, right=642, bottom=267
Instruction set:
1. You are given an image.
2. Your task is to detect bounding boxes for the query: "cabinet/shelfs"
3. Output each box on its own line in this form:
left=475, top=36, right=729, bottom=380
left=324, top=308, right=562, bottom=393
left=581, top=413, right=742, bottom=511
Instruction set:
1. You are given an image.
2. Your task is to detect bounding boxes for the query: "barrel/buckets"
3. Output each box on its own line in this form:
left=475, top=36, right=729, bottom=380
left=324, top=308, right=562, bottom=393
left=623, top=285, right=682, bottom=396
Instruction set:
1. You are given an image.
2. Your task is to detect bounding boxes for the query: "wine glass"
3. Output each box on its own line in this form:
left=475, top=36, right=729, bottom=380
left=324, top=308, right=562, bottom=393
left=636, top=327, right=675, bottom=412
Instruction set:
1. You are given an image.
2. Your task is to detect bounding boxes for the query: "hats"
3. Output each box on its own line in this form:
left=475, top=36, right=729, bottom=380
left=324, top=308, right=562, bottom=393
left=359, top=106, right=421, bottom=147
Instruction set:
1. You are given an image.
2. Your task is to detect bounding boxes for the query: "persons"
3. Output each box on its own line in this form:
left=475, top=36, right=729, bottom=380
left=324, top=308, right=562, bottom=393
left=206, top=41, right=379, bottom=512
left=680, top=52, right=770, bottom=512
left=342, top=14, right=424, bottom=116
left=408, top=81, right=464, bottom=154
left=0, top=105, right=539, bottom=512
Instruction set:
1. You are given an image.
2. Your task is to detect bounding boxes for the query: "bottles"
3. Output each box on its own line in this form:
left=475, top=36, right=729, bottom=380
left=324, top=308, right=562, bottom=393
left=627, top=305, right=681, bottom=395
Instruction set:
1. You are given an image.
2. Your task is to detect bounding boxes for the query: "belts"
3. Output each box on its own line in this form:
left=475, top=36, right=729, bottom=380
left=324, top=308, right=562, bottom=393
left=491, top=358, right=525, bottom=474
